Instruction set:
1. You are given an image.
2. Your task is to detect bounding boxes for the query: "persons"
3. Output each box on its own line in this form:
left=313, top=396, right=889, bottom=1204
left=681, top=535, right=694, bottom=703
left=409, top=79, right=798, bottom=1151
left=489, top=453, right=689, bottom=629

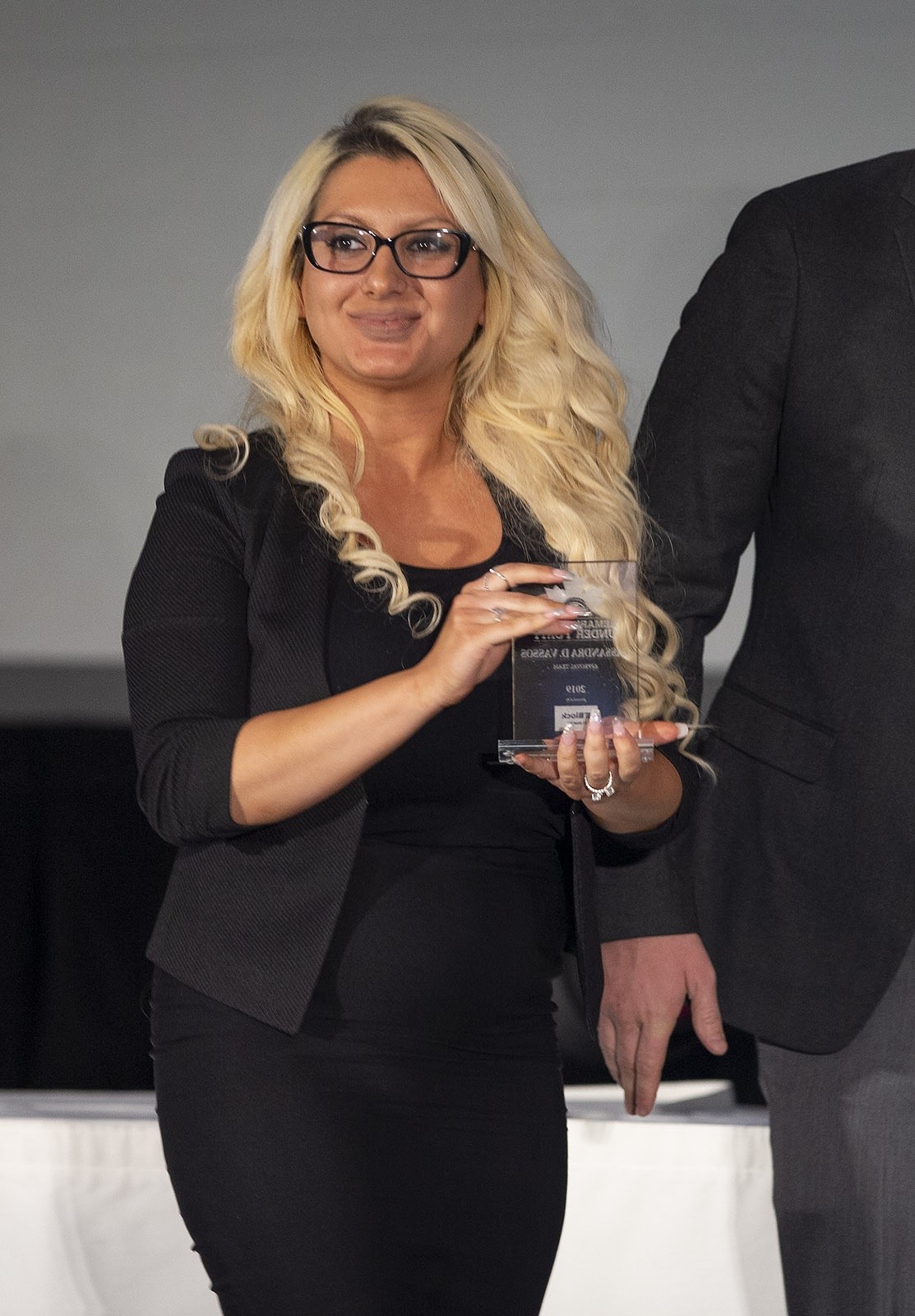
left=120, top=95, right=719, bottom=1316
left=583, top=149, right=915, bottom=1316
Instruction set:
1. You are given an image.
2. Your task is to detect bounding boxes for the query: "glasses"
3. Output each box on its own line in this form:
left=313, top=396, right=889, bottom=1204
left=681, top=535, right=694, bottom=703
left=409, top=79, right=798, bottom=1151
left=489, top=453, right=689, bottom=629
left=301, top=221, right=473, bottom=282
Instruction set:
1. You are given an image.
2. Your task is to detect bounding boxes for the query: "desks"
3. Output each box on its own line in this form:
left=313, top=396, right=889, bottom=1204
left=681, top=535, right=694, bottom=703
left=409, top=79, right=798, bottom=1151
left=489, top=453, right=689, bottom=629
left=0, top=1081, right=789, bottom=1316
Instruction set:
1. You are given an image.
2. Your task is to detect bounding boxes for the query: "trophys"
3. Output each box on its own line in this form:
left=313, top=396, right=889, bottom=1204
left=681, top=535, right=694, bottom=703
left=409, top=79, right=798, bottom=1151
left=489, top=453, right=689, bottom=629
left=497, top=560, right=654, bottom=764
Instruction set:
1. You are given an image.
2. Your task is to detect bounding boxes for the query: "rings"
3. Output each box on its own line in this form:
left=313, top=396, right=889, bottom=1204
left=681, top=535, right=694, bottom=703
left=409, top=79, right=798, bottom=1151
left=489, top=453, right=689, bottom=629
left=583, top=771, right=616, bottom=802
left=483, top=568, right=512, bottom=592
left=488, top=606, right=507, bottom=623
